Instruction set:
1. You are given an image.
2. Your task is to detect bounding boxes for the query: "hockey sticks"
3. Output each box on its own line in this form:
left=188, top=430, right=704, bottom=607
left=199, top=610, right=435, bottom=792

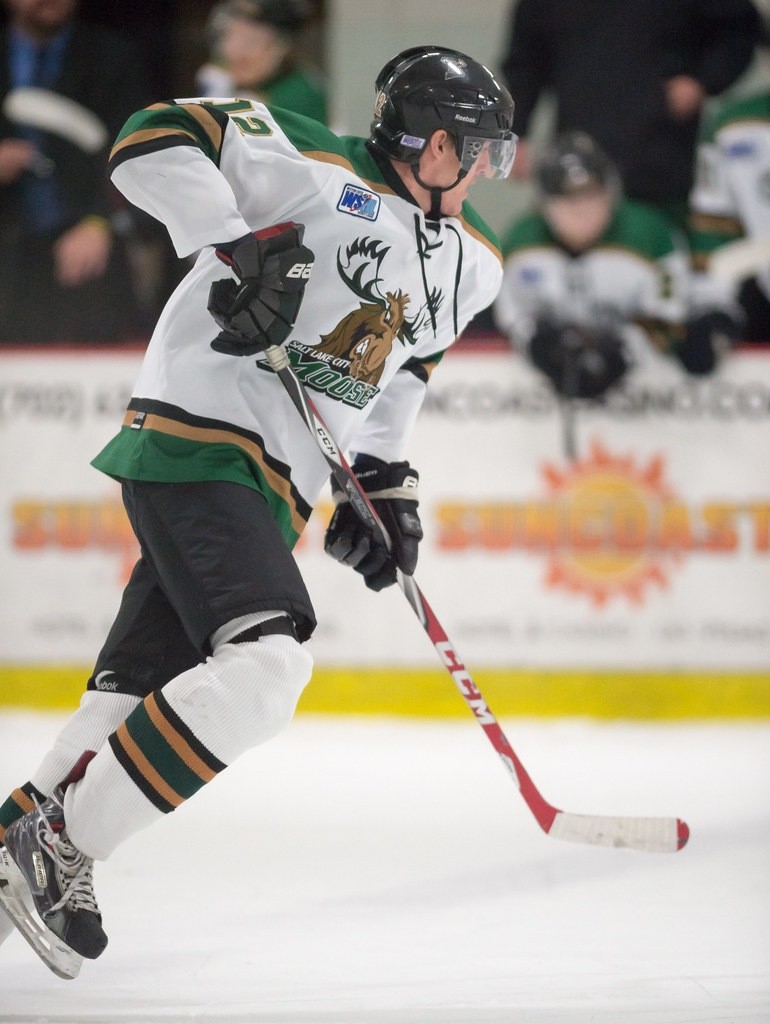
left=266, top=343, right=693, bottom=853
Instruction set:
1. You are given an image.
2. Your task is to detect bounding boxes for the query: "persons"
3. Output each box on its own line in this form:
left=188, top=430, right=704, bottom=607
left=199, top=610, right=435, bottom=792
left=0, top=46, right=520, bottom=982
left=0, top=0, right=327, bottom=347
left=501, top=0, right=770, bottom=397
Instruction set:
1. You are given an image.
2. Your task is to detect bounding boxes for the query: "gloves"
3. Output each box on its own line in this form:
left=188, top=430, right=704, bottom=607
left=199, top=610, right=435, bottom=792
left=529, top=315, right=629, bottom=399
left=207, top=220, right=315, bottom=357
left=323, top=452, right=423, bottom=592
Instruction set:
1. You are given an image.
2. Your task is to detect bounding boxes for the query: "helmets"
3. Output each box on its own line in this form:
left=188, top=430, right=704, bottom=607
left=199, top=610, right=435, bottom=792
left=531, top=132, right=617, bottom=200
left=223, top=0, right=323, bottom=34
left=368, top=46, right=519, bottom=181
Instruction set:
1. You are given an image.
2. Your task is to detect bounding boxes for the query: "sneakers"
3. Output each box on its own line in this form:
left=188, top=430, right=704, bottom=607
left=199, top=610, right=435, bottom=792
left=0, top=750, right=108, bottom=979
